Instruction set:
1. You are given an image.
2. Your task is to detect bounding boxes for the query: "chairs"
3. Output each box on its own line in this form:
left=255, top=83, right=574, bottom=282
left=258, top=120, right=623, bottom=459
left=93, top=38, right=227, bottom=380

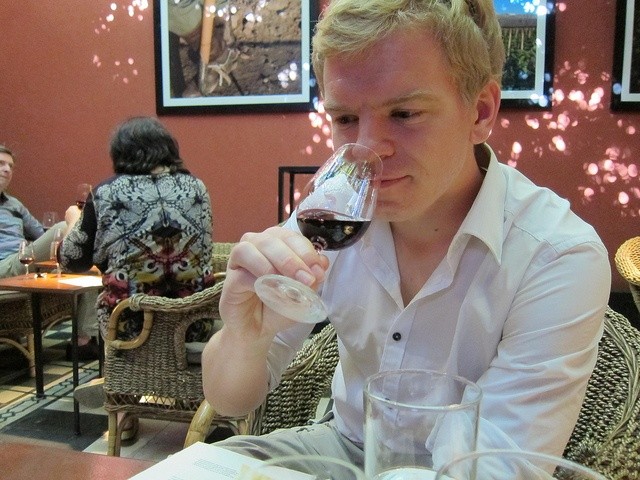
left=182, top=305, right=640, bottom=479
left=213, top=240, right=237, bottom=275
left=612, top=235, right=640, bottom=312
left=0, top=290, right=73, bottom=377
left=101, top=242, right=249, bottom=458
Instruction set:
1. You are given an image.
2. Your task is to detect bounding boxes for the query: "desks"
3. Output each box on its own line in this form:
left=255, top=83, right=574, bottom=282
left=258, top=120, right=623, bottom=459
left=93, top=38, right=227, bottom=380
left=30, top=260, right=101, bottom=398
left=0, top=270, right=105, bottom=436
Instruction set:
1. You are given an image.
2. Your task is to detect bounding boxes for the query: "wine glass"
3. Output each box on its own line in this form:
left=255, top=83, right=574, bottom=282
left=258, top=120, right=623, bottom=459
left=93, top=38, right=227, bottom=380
left=254, top=143, right=383, bottom=323
left=49, top=241, right=65, bottom=279
left=43, top=211, right=55, bottom=233
left=17, top=239, right=35, bottom=279
left=53, top=229, right=65, bottom=242
left=75, top=182, right=90, bottom=211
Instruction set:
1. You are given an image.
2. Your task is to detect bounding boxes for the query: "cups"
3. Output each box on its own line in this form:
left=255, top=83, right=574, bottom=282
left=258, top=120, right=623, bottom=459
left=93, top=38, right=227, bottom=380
left=361, top=368, right=483, bottom=480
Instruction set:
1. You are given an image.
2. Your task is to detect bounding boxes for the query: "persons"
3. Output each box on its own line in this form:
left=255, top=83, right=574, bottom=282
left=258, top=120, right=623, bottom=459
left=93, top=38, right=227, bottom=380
left=0, top=146, right=95, bottom=281
left=163, top=0, right=217, bottom=99
left=56, top=115, right=213, bottom=443
left=201, top=1, right=612, bottom=480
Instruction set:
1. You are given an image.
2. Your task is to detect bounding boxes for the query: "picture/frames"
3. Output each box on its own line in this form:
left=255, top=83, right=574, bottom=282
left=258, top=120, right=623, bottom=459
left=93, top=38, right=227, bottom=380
left=489, top=0, right=556, bottom=109
left=609, top=0, right=640, bottom=112
left=152, top=0, right=320, bottom=117
